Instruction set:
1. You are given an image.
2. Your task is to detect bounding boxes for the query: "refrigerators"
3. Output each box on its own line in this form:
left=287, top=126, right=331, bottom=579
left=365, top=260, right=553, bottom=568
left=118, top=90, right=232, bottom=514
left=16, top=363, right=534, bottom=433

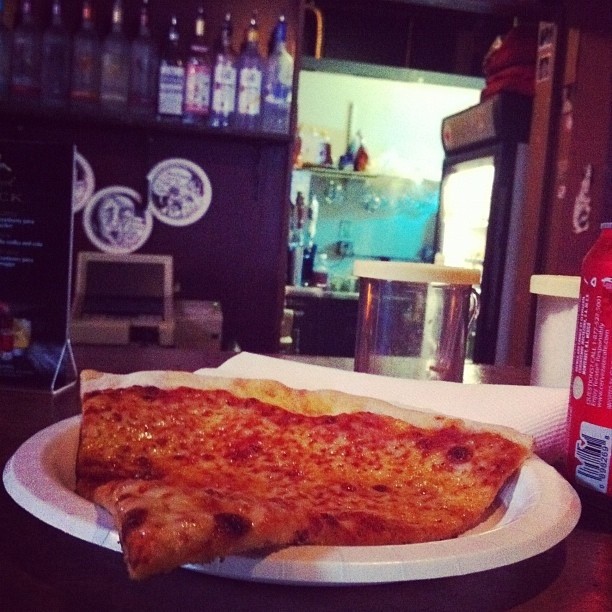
left=420, top=95, right=533, bottom=366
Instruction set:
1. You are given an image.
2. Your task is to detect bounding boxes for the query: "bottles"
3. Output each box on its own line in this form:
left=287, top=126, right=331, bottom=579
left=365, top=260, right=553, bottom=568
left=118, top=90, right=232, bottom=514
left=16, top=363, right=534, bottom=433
left=262, top=16, right=294, bottom=137
left=157, top=14, right=184, bottom=119
left=12, top=1, right=43, bottom=104
left=237, top=12, right=265, bottom=134
left=181, top=4, right=212, bottom=126
left=100, top=0, right=130, bottom=111
left=129, top=1, right=157, bottom=112
left=69, top=0, right=98, bottom=106
left=207, top=13, right=239, bottom=129
left=41, top=0, right=73, bottom=106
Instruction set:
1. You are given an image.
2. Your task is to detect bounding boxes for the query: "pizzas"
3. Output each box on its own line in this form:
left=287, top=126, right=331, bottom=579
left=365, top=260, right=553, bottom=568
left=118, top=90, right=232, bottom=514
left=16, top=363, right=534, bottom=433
left=74, top=370, right=534, bottom=586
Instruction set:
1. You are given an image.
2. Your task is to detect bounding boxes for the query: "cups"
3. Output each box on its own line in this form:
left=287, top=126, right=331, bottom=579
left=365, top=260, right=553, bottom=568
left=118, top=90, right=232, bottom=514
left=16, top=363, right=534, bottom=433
left=353, top=277, right=481, bottom=383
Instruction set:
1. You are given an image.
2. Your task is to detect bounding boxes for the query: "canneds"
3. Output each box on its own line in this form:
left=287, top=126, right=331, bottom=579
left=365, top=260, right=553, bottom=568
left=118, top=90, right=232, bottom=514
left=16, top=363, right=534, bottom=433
left=566, top=223, right=612, bottom=533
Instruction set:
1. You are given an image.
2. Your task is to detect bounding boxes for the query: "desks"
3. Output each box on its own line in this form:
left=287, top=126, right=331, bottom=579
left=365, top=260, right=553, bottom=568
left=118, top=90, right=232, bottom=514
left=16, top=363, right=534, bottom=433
left=1, top=347, right=612, bottom=612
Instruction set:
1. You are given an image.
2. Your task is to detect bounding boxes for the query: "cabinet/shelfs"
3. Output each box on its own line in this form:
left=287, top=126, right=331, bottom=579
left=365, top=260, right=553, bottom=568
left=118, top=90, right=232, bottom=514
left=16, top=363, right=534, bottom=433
left=0, top=0, right=304, bottom=351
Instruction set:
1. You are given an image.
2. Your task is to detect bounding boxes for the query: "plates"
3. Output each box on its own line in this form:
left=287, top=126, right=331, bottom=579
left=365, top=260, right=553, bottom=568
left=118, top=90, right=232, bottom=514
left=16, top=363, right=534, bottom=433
left=3, top=411, right=581, bottom=587
